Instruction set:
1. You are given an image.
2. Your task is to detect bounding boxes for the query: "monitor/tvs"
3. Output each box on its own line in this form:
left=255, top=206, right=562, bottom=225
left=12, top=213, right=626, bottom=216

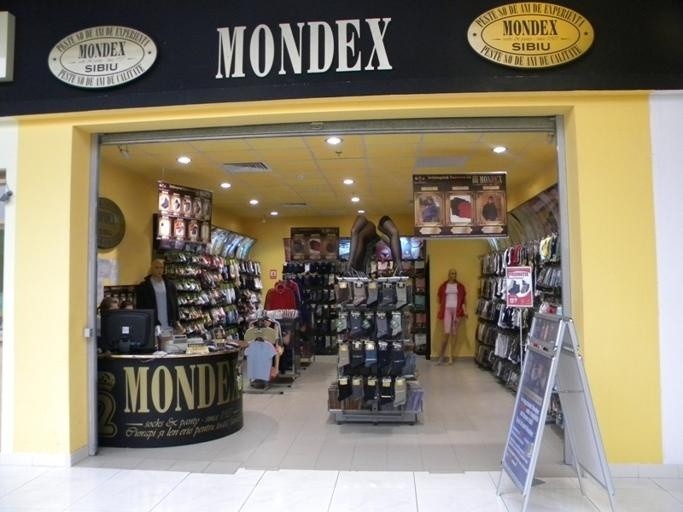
left=101, top=309, right=156, bottom=352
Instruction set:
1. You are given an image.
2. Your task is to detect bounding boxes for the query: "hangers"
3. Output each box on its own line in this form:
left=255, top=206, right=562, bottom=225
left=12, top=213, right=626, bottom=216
left=251, top=316, right=279, bottom=342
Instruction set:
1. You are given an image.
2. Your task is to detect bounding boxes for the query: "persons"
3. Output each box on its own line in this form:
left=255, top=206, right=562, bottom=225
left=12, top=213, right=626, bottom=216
left=118, top=299, right=135, bottom=309
left=482, top=195, right=498, bottom=221
left=136, top=258, right=184, bottom=334
left=346, top=216, right=403, bottom=276
left=98, top=296, right=120, bottom=314
left=434, top=267, right=469, bottom=364
